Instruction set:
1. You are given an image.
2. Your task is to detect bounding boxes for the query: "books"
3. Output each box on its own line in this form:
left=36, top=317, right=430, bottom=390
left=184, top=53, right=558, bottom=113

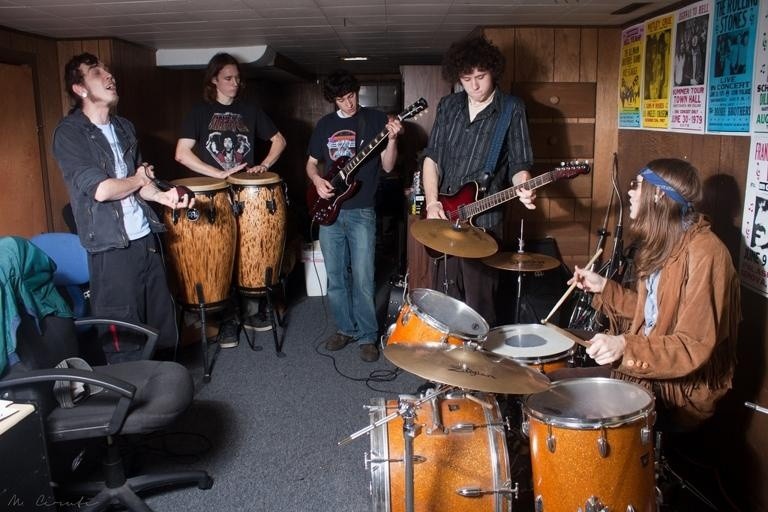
left=0, top=399, right=35, bottom=435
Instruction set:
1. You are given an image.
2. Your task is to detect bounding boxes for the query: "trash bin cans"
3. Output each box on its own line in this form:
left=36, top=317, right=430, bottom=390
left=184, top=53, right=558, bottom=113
left=300, top=241, right=327, bottom=297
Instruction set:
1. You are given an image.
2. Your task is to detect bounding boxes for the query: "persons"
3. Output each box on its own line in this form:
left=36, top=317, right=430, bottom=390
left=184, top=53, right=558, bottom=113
left=417, top=36, right=537, bottom=321
left=714, top=31, right=749, bottom=77
left=174, top=53, right=286, bottom=348
left=305, top=70, right=403, bottom=362
left=546, top=158, right=742, bottom=436
left=51, top=51, right=196, bottom=365
left=675, top=34, right=704, bottom=85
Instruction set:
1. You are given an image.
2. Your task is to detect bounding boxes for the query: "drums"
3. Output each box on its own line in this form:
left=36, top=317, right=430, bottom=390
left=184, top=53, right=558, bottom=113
left=484, top=324, right=578, bottom=373
left=522, top=377, right=663, bottom=512
left=162, top=177, right=237, bottom=305
left=364, top=391, right=519, bottom=512
left=385, top=288, right=490, bottom=349
left=227, top=171, right=288, bottom=291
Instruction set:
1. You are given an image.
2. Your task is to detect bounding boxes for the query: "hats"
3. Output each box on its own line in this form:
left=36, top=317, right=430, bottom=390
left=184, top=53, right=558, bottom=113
left=52, top=357, right=102, bottom=407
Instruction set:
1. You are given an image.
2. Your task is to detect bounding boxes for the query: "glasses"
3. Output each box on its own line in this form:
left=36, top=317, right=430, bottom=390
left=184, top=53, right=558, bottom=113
left=630, top=178, right=642, bottom=189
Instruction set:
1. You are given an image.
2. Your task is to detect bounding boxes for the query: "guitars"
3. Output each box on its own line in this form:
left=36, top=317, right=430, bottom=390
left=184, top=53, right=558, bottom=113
left=306, top=96, right=429, bottom=226
left=419, top=160, right=591, bottom=259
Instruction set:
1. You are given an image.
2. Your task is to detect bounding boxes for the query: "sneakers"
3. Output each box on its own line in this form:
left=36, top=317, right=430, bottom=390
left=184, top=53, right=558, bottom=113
left=360, top=343, right=378, bottom=362
left=242, top=316, right=272, bottom=332
left=325, top=333, right=352, bottom=351
left=218, top=322, right=238, bottom=348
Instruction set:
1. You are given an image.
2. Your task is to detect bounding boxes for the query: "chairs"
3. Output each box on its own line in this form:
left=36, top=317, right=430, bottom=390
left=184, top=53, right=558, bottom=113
left=28, top=233, right=92, bottom=320
left=0, top=238, right=212, bottom=512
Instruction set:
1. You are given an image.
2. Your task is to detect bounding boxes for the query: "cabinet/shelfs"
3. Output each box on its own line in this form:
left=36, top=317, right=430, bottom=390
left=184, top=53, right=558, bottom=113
left=0, top=398, right=57, bottom=511
left=509, top=82, right=596, bottom=282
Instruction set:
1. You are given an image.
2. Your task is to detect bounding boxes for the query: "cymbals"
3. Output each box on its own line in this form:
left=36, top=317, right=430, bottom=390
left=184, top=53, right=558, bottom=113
left=380, top=339, right=551, bottom=394
left=410, top=217, right=499, bottom=258
left=488, top=251, right=560, bottom=271
